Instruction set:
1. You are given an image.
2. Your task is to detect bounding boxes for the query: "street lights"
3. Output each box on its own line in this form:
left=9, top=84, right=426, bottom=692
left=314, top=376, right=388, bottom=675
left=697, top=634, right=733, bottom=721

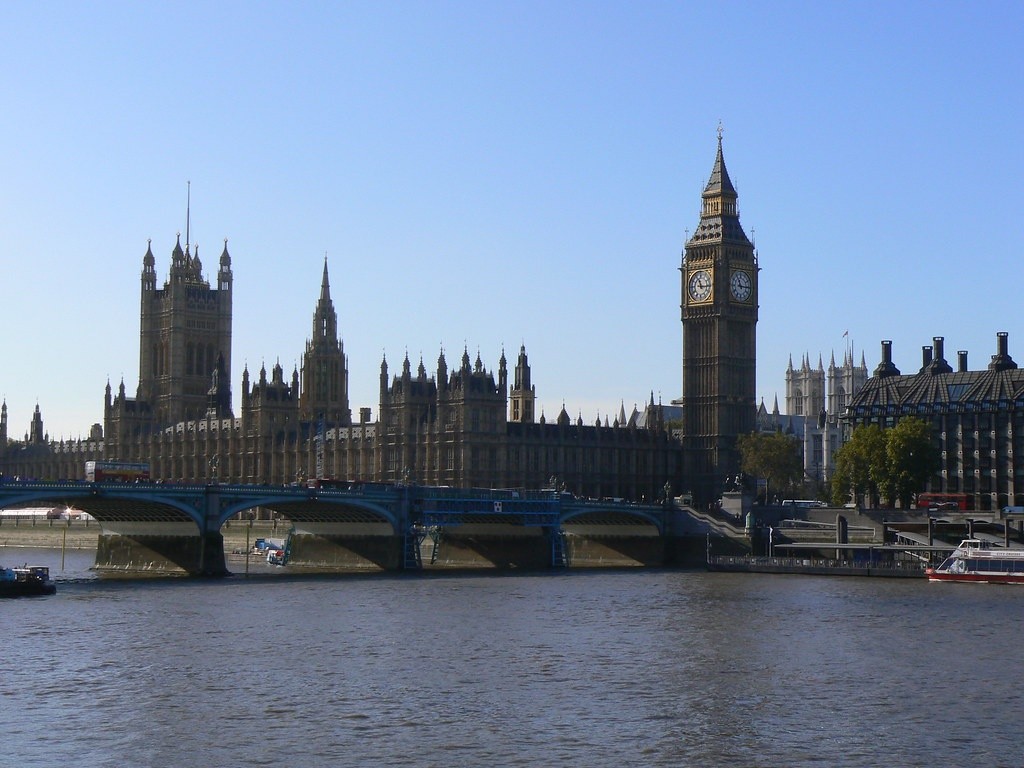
left=209, top=454, right=221, bottom=487
left=816, top=462, right=818, bottom=488
left=1004, top=518, right=1014, bottom=547
left=929, top=518, right=936, bottom=560
left=966, top=519, right=974, bottom=540
left=400, top=465, right=411, bottom=487
left=663, top=480, right=670, bottom=504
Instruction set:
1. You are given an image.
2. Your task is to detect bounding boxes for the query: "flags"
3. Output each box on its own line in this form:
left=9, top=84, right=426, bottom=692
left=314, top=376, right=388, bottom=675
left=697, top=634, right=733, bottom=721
left=843, top=331, right=848, bottom=337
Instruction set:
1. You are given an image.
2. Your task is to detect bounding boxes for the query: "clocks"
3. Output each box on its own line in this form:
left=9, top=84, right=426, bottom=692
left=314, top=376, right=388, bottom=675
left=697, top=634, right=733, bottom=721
left=729, top=269, right=753, bottom=304
left=688, top=269, right=712, bottom=303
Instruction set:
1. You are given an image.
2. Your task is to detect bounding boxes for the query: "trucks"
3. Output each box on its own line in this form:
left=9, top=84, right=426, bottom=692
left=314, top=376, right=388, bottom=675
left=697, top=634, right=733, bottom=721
left=1002, top=507, right=1024, bottom=512
left=842, top=504, right=856, bottom=508
left=917, top=492, right=967, bottom=510
left=603, top=497, right=624, bottom=503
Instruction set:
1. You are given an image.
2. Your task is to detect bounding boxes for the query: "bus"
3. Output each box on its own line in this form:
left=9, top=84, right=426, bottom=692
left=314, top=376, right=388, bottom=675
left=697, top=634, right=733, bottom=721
left=304, top=477, right=574, bottom=502
left=84, top=461, right=150, bottom=486
left=782, top=499, right=828, bottom=507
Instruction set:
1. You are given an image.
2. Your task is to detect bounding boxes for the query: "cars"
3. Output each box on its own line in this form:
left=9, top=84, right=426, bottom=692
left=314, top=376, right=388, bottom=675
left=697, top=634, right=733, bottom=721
left=933, top=519, right=952, bottom=524
left=588, top=498, right=598, bottom=501
left=974, top=520, right=989, bottom=524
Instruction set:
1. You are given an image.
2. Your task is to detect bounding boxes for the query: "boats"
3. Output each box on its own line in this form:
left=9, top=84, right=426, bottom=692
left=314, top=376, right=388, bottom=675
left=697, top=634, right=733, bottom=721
left=0, top=564, right=57, bottom=600
left=267, top=550, right=290, bottom=568
left=928, top=538, right=1024, bottom=585
